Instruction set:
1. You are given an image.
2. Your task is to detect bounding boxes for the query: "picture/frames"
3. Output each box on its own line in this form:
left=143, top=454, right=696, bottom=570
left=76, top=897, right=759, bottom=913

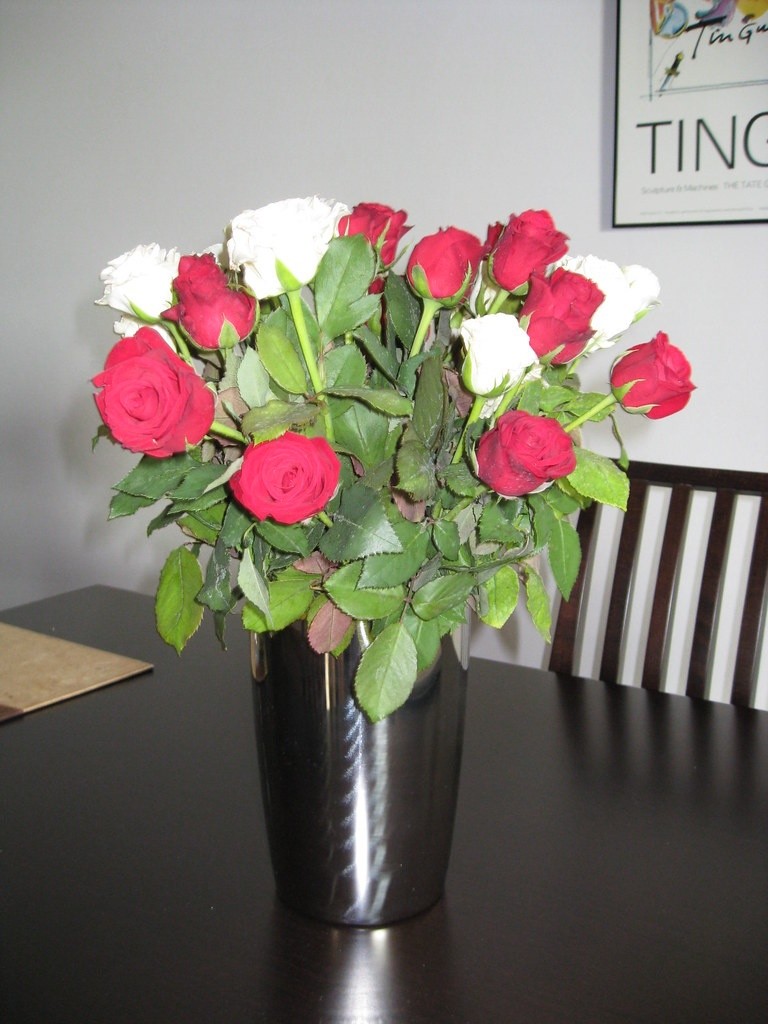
left=611, top=0, right=768, bottom=228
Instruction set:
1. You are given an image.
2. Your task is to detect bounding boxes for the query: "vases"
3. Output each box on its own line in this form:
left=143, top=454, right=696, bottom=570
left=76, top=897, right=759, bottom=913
left=242, top=613, right=465, bottom=922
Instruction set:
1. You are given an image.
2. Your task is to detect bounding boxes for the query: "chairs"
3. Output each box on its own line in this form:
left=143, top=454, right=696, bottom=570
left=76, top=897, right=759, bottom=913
left=548, top=458, right=768, bottom=711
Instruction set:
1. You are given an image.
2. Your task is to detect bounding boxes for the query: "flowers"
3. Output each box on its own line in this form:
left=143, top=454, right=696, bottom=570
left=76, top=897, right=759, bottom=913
left=90, top=195, right=700, bottom=721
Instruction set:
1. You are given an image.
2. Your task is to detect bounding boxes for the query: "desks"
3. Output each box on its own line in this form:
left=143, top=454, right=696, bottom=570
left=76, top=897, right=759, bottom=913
left=0, top=585, right=768, bottom=1024
